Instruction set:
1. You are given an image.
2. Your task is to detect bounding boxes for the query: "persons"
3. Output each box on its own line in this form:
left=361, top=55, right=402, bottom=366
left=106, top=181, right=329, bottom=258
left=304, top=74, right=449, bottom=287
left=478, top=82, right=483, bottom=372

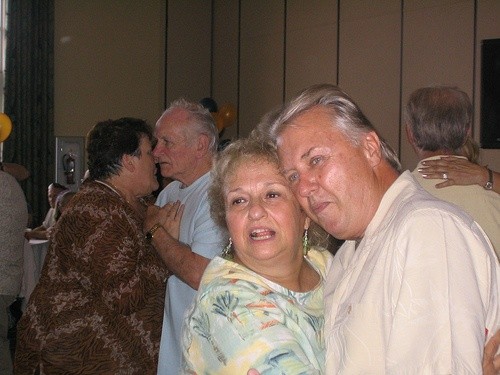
left=24, top=183, right=77, bottom=240
left=255, top=84, right=500, bottom=375
left=0, top=170, right=29, bottom=375
left=15, top=118, right=184, bottom=375
left=181, top=137, right=500, bottom=375
left=406, top=88, right=500, bottom=261
left=144, top=101, right=233, bottom=375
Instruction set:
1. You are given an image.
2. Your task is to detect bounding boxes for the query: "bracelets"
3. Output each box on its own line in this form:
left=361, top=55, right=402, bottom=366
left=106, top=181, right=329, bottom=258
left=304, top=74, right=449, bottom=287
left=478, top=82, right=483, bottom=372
left=25, top=232, right=27, bottom=238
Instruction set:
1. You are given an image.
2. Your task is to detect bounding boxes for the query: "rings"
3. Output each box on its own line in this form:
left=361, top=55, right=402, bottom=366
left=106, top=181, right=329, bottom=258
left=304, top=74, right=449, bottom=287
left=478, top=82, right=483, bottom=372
left=443, top=171, right=447, bottom=179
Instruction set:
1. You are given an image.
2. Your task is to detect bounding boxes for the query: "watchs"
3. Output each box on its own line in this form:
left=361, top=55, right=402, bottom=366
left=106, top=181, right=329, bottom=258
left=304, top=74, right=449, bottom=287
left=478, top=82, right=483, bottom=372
left=484, top=168, right=493, bottom=190
left=144, top=224, right=162, bottom=242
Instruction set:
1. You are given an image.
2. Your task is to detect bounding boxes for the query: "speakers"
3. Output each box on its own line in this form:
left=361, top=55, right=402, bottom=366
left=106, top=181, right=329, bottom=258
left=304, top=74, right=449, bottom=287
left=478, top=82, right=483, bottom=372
left=479, top=38, right=500, bottom=150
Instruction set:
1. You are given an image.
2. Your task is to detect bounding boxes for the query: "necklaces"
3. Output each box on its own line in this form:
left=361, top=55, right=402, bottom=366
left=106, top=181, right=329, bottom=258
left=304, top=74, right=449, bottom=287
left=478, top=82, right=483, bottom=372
left=95, top=180, right=121, bottom=197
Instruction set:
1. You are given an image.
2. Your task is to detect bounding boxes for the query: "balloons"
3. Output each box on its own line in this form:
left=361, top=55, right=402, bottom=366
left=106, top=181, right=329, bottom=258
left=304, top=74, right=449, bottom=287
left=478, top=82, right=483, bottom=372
left=0, top=112, right=12, bottom=142
left=201, top=97, right=217, bottom=113
left=209, top=112, right=224, bottom=131
left=218, top=105, right=236, bottom=126
